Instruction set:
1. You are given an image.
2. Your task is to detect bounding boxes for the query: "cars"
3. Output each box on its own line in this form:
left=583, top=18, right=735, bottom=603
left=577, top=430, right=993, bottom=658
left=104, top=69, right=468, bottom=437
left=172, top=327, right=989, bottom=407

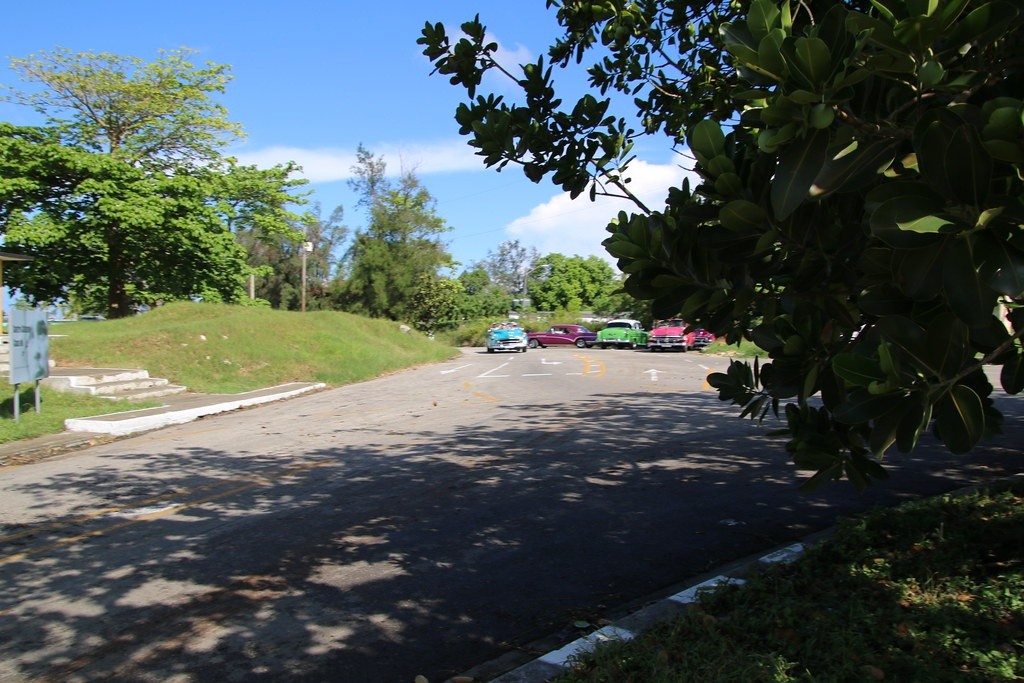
left=596, top=318, right=649, bottom=349
left=646, top=318, right=716, bottom=352
left=527, top=323, right=597, bottom=348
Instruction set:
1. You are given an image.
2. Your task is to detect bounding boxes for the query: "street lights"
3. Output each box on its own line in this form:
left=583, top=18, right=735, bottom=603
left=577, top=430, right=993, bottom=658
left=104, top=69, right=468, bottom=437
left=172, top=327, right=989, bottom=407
left=299, top=240, right=313, bottom=312
left=520, top=263, right=552, bottom=307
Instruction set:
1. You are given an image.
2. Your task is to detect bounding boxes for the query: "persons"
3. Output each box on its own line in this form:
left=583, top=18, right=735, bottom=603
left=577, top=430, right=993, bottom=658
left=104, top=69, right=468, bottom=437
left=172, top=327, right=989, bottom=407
left=490, top=322, right=564, bottom=333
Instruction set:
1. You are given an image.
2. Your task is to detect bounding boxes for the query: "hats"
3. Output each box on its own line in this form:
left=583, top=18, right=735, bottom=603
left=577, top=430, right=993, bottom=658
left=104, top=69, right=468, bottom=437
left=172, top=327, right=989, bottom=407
left=493, top=323, right=496, bottom=325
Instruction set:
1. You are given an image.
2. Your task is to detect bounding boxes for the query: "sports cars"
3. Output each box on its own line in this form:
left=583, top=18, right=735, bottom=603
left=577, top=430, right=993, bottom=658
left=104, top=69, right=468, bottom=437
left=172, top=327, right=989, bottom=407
left=485, top=326, right=529, bottom=354
